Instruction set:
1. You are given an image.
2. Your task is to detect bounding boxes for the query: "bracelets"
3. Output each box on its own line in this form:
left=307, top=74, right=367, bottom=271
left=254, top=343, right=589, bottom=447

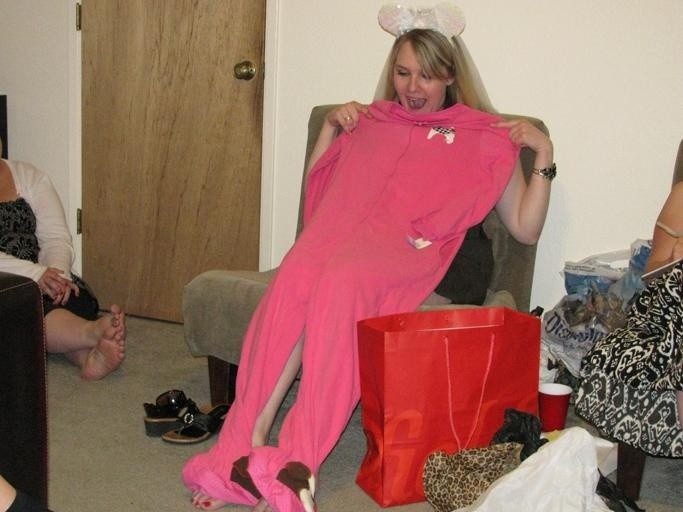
left=531, top=163, right=556, bottom=182
left=188, top=3, right=557, bottom=512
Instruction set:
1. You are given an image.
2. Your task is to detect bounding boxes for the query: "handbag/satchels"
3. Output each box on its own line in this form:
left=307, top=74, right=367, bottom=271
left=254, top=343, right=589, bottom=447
left=357, top=307, right=541, bottom=508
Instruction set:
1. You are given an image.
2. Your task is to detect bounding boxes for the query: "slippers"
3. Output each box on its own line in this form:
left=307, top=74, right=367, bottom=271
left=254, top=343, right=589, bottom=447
left=144, top=390, right=231, bottom=443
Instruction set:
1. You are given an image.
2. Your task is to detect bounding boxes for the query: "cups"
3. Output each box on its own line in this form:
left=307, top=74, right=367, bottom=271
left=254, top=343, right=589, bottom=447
left=538, top=382, right=574, bottom=433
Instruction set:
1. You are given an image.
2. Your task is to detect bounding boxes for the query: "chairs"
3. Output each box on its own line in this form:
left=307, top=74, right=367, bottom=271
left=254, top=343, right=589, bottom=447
left=181, top=104, right=552, bottom=422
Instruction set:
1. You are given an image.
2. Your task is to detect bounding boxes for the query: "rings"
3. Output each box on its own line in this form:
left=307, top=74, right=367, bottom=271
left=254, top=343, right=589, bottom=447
left=341, top=113, right=353, bottom=125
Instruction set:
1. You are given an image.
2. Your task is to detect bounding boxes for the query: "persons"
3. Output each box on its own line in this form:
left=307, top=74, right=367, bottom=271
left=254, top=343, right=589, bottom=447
left=0, top=138, right=126, bottom=381
left=574, top=137, right=683, bottom=460
left=0, top=475, right=49, bottom=512
